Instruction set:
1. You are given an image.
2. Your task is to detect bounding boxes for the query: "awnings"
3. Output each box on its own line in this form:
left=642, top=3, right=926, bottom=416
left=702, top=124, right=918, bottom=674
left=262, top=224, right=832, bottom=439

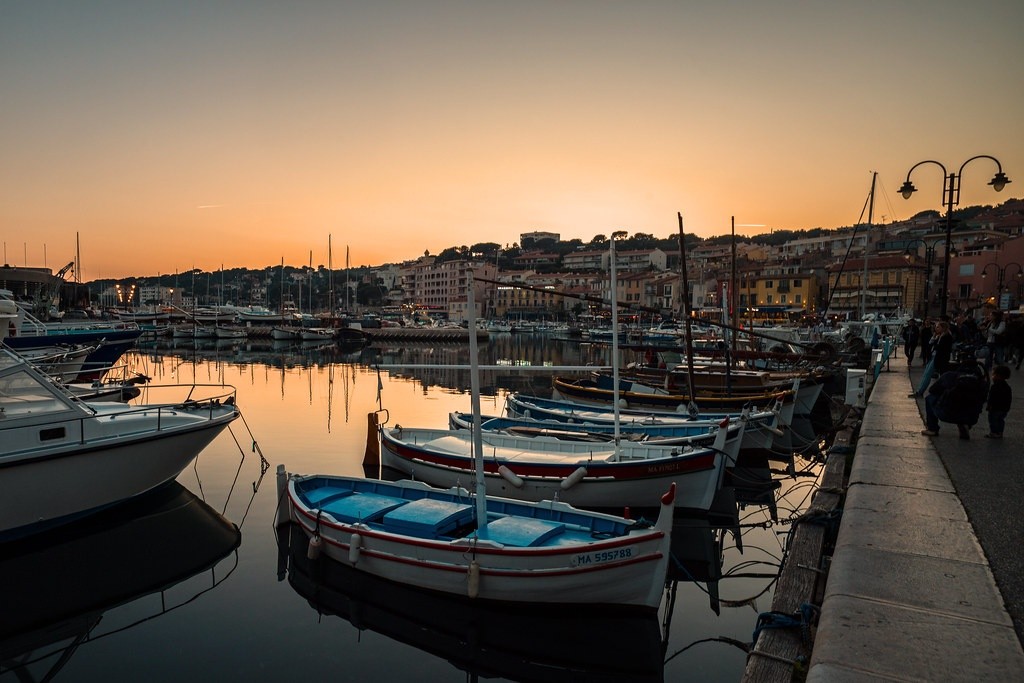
left=816, top=310, right=856, bottom=315
left=784, top=308, right=806, bottom=312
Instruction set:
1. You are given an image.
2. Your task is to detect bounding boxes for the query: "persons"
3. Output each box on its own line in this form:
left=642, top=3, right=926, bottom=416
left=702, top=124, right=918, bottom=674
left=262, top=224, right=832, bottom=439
left=10, top=321, right=16, bottom=337
left=922, top=354, right=987, bottom=439
left=908, top=322, right=953, bottom=398
left=985, top=366, right=1013, bottom=438
left=939, top=311, right=1024, bottom=371
left=918, top=315, right=934, bottom=365
left=817, top=320, right=824, bottom=334
left=902, top=319, right=920, bottom=365
left=827, top=317, right=831, bottom=329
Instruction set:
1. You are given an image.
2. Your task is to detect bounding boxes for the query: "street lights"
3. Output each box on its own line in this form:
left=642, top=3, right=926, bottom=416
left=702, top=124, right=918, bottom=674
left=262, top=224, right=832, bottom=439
left=903, top=237, right=957, bottom=318
left=896, top=155, right=1012, bottom=318
left=982, top=261, right=1023, bottom=315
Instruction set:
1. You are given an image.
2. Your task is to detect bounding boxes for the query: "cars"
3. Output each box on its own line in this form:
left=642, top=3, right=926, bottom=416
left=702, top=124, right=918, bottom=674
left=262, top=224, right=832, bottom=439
left=49, top=305, right=97, bottom=322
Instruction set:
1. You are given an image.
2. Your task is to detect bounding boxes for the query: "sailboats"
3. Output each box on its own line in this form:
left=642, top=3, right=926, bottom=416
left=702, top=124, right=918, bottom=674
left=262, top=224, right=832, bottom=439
left=139, top=232, right=372, bottom=352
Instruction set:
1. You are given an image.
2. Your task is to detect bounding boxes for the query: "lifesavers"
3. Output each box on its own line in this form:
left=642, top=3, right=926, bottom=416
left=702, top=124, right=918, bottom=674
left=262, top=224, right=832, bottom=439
left=848, top=337, right=865, bottom=346
left=849, top=335, right=858, bottom=339
left=162, top=306, right=175, bottom=312
left=768, top=342, right=793, bottom=354
left=845, top=333, right=852, bottom=341
left=809, top=342, right=838, bottom=357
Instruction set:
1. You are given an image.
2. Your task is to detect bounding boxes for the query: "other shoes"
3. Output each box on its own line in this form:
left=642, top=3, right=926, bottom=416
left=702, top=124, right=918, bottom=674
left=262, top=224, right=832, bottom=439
left=907, top=391, right=923, bottom=400
left=921, top=428, right=939, bottom=437
left=985, top=432, right=1004, bottom=438
left=908, top=357, right=912, bottom=366
left=922, top=362, right=925, bottom=366
left=959, top=434, right=970, bottom=441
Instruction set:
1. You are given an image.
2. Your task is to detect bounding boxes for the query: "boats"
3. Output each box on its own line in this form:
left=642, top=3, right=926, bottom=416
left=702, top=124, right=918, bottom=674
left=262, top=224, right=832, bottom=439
left=0, top=281, right=144, bottom=382
left=275, top=170, right=906, bottom=611
left=276, top=522, right=668, bottom=683
left=0, top=337, right=239, bottom=543
left=0, top=481, right=242, bottom=683
